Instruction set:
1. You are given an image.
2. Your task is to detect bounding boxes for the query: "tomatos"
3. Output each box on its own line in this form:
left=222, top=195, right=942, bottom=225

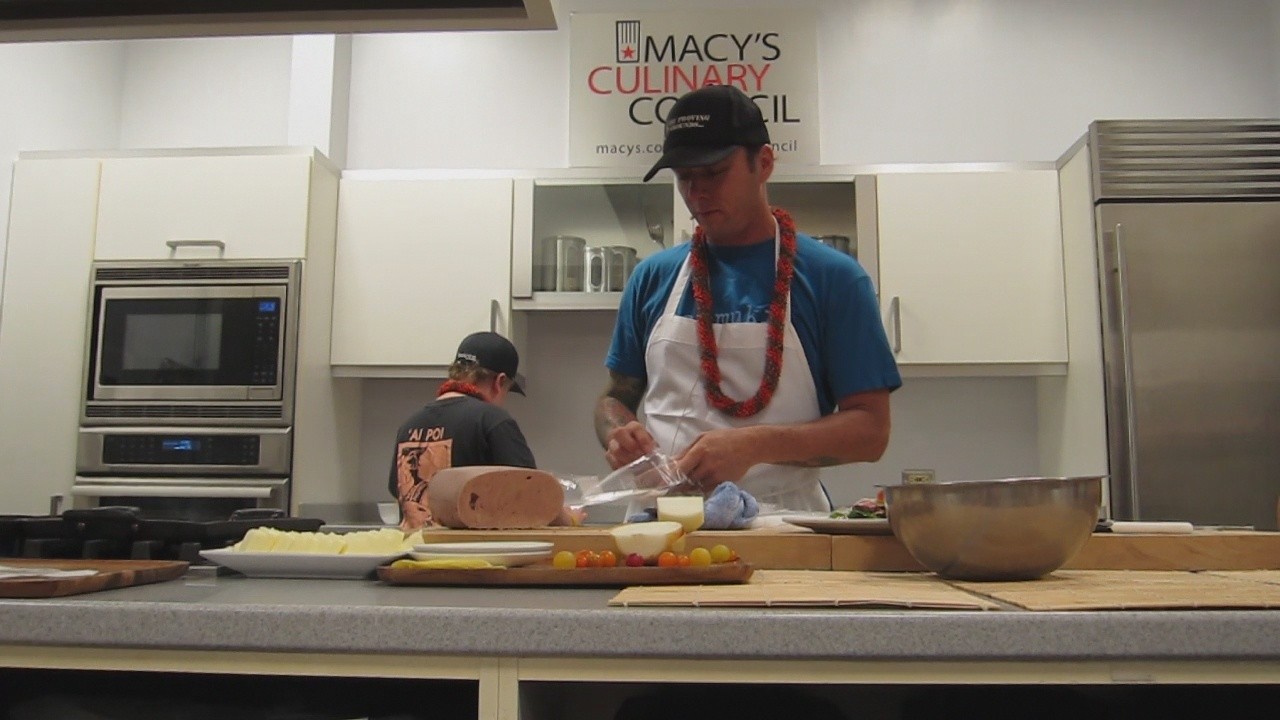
left=575, top=550, right=688, bottom=570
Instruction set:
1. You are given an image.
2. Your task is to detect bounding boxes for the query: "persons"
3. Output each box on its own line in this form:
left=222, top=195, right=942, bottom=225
left=597, top=83, right=904, bottom=512
left=388, top=331, right=587, bottom=525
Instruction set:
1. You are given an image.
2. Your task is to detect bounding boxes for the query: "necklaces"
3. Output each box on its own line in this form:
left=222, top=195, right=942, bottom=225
left=689, top=206, right=797, bottom=418
left=436, top=379, right=491, bottom=403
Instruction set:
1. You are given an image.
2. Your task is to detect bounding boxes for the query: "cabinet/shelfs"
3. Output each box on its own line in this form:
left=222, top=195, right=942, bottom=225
left=516, top=173, right=877, bottom=334
left=328, top=166, right=515, bottom=383
left=870, top=165, right=1067, bottom=376
left=0, top=140, right=361, bottom=517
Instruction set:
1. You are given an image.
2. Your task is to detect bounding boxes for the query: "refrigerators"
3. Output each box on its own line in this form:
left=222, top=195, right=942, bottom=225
left=1034, top=118, right=1280, bottom=533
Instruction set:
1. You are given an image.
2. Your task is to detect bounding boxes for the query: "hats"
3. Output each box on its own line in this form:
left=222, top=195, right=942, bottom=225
left=455, top=332, right=527, bottom=397
left=644, top=84, right=769, bottom=182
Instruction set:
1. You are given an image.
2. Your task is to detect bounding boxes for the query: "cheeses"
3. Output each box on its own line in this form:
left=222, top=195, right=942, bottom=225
left=656, top=496, right=703, bottom=533
left=609, top=521, right=683, bottom=559
left=237, top=526, right=421, bottom=555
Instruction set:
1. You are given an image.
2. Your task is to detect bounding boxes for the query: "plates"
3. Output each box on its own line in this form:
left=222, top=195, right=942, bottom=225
left=375, top=554, right=757, bottom=586
left=404, top=542, right=553, bottom=568
left=782, top=516, right=892, bottom=536
left=198, top=547, right=411, bottom=580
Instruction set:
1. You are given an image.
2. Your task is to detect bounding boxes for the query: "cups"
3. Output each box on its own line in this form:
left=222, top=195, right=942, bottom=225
left=583, top=244, right=636, bottom=292
left=814, top=234, right=849, bottom=254
left=540, top=235, right=587, bottom=292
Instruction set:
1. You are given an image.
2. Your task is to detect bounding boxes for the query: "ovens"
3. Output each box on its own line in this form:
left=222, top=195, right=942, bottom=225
left=70, top=261, right=301, bottom=518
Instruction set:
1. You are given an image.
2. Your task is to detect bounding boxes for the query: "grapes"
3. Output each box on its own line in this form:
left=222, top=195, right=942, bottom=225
left=553, top=551, right=576, bottom=570
left=689, top=544, right=730, bottom=566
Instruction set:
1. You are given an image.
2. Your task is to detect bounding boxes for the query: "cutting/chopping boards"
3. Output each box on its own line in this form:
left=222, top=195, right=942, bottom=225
left=0, top=555, right=190, bottom=598
left=422, top=524, right=833, bottom=571
left=830, top=533, right=1280, bottom=572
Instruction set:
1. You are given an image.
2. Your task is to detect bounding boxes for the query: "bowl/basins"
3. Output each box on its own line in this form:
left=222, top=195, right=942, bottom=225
left=874, top=472, right=1111, bottom=583
left=377, top=503, right=399, bottom=525
